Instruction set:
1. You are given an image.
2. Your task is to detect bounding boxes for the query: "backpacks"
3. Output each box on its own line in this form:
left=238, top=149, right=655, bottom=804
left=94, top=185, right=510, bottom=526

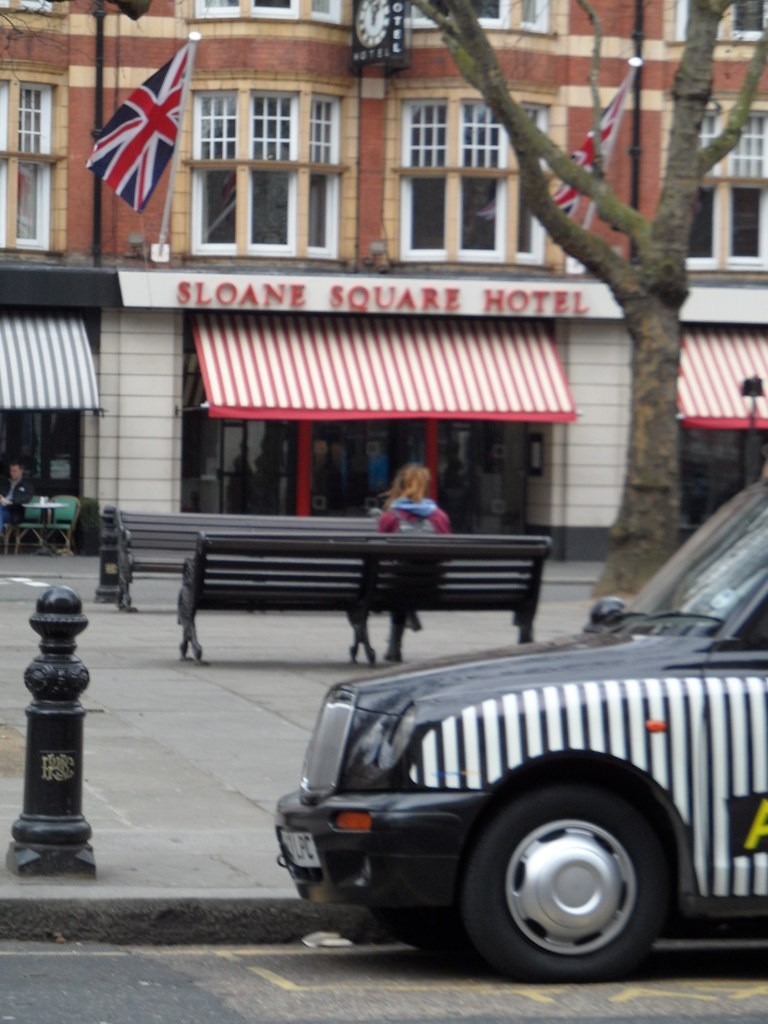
left=389, top=509, right=435, bottom=533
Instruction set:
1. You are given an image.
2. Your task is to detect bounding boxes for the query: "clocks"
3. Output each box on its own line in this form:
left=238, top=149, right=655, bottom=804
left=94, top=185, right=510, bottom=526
left=350, top=0, right=410, bottom=78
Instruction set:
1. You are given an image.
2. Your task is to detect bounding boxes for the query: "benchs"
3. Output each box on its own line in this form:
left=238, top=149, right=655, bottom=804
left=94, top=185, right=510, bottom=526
left=174, top=527, right=553, bottom=670
left=111, top=507, right=381, bottom=612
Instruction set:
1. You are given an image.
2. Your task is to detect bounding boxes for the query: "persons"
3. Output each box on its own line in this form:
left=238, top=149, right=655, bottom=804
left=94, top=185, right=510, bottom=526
left=1, top=459, right=35, bottom=536
left=378, top=462, right=450, bottom=662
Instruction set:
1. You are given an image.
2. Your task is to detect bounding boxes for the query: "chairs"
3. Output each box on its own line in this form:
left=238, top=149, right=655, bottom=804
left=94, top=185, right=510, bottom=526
left=14, top=495, right=46, bottom=555
left=44, top=495, right=82, bottom=557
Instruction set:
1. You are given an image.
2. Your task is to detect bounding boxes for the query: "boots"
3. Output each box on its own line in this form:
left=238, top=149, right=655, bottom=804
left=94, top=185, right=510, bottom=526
left=406, top=613, right=422, bottom=631
left=384, top=620, right=405, bottom=661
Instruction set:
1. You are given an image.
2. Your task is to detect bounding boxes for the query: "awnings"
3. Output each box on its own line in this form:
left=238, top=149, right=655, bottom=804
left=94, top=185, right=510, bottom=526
left=0, top=310, right=103, bottom=416
left=185, top=309, right=575, bottom=422
left=673, top=323, right=766, bottom=431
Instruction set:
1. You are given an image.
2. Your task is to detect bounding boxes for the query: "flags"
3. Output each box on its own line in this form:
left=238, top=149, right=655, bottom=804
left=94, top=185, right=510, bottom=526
left=221, top=170, right=236, bottom=220
left=83, top=38, right=192, bottom=213
left=547, top=73, right=632, bottom=218
left=475, top=199, right=495, bottom=225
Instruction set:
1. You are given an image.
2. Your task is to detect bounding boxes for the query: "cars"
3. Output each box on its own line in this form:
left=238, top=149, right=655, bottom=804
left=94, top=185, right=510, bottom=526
left=274, top=475, right=768, bottom=985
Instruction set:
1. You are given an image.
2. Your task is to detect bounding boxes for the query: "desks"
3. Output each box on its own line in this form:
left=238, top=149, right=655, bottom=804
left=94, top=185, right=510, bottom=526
left=22, top=502, right=69, bottom=559
